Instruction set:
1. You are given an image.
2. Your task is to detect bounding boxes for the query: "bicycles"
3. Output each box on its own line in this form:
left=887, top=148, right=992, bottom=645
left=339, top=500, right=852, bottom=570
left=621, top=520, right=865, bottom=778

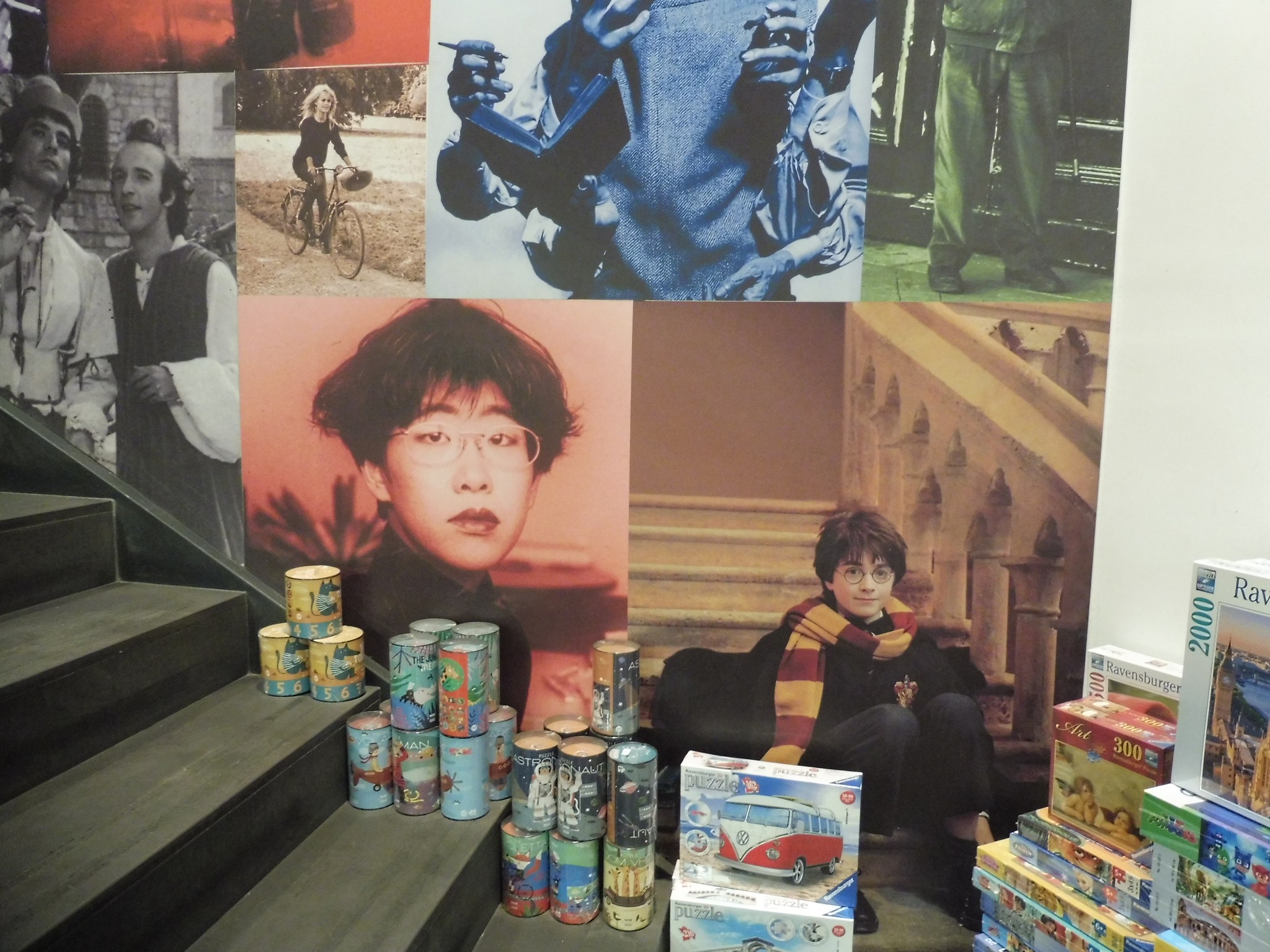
left=281, top=165, right=365, bottom=278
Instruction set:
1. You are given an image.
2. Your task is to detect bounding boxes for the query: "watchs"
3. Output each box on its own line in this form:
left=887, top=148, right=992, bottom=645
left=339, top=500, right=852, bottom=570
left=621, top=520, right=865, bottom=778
left=808, top=62, right=855, bottom=84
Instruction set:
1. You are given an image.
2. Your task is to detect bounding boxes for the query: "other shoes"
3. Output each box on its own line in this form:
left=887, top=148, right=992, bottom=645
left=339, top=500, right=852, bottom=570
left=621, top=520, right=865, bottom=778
left=321, top=242, right=331, bottom=254
left=950, top=862, right=984, bottom=931
left=298, top=204, right=307, bottom=220
left=853, top=892, right=878, bottom=932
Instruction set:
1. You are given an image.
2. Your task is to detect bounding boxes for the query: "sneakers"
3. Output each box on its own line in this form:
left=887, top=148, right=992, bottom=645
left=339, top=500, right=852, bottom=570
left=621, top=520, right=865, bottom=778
left=928, top=266, right=965, bottom=294
left=1005, top=262, right=1065, bottom=294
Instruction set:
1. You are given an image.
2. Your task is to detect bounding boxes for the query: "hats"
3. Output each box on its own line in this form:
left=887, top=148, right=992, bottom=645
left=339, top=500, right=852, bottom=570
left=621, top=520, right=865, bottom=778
left=3, top=76, right=84, bottom=142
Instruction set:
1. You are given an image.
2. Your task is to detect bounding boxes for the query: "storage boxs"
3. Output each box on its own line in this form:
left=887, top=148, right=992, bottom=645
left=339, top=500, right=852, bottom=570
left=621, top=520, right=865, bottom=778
left=669, top=750, right=863, bottom=952
left=971, top=559, right=1270, bottom=952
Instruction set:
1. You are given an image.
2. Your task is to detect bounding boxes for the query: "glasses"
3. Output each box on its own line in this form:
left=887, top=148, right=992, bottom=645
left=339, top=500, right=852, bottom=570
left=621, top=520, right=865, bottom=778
left=834, top=566, right=894, bottom=584
left=388, top=421, right=541, bottom=470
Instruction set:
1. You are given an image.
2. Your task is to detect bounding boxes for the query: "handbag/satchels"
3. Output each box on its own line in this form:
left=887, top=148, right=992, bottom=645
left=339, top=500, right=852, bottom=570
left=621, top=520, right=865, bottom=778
left=341, top=168, right=372, bottom=192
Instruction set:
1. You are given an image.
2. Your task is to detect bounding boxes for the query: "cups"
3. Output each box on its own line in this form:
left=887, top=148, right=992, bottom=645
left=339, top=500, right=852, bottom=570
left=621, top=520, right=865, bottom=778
left=378, top=618, right=517, bottom=821
left=284, top=565, right=342, bottom=639
left=590, top=638, right=641, bottom=735
left=500, top=713, right=658, bottom=932
left=258, top=622, right=310, bottom=698
left=345, top=711, right=394, bottom=810
left=310, top=626, right=366, bottom=702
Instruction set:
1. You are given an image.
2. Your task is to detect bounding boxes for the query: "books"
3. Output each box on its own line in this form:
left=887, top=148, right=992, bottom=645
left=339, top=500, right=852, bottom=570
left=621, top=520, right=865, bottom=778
left=466, top=73, right=630, bottom=200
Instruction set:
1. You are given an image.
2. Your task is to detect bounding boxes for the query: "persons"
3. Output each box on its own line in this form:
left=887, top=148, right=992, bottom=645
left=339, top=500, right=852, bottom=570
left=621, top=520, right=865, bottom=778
left=1062, top=777, right=1141, bottom=846
left=0, top=75, right=243, bottom=569
left=310, top=299, right=583, bottom=734
left=292, top=84, right=355, bottom=253
left=437, top=0, right=866, bottom=301
left=733, top=510, right=996, bottom=931
left=929, top=0, right=1074, bottom=293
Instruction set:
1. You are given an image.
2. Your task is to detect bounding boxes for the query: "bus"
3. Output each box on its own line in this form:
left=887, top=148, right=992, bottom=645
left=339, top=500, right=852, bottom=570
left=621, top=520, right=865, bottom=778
left=713, top=794, right=843, bottom=885
